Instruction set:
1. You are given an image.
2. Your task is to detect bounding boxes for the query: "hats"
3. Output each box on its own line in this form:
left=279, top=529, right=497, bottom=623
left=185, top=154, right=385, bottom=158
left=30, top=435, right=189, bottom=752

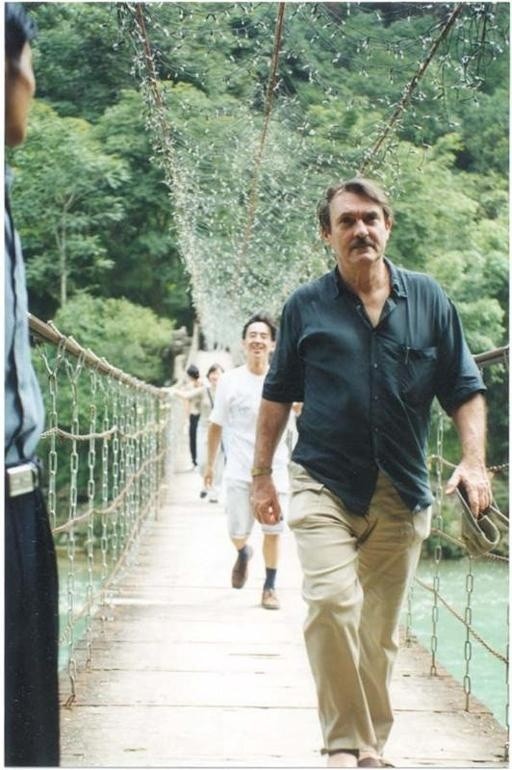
left=454, top=486, right=507, bottom=557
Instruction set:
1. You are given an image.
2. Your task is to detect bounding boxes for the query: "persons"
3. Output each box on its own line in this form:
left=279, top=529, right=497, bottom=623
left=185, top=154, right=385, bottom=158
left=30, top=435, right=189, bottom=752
left=203, top=312, right=303, bottom=614
left=249, top=177, right=496, bottom=768
left=183, top=364, right=206, bottom=468
left=6, top=1, right=62, bottom=767
left=160, top=362, right=227, bottom=504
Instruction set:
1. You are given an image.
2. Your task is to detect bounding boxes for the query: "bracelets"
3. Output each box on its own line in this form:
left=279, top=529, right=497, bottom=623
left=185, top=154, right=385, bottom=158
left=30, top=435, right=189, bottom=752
left=250, top=467, right=273, bottom=477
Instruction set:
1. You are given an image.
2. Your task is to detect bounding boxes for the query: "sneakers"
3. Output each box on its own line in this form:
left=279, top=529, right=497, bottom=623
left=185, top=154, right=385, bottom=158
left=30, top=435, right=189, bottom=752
left=327, top=749, right=386, bottom=768
left=232, top=546, right=252, bottom=588
left=262, top=588, right=280, bottom=609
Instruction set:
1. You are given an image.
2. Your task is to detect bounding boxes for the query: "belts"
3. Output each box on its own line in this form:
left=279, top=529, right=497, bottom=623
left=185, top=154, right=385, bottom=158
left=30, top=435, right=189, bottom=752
left=6, top=460, right=42, bottom=497
